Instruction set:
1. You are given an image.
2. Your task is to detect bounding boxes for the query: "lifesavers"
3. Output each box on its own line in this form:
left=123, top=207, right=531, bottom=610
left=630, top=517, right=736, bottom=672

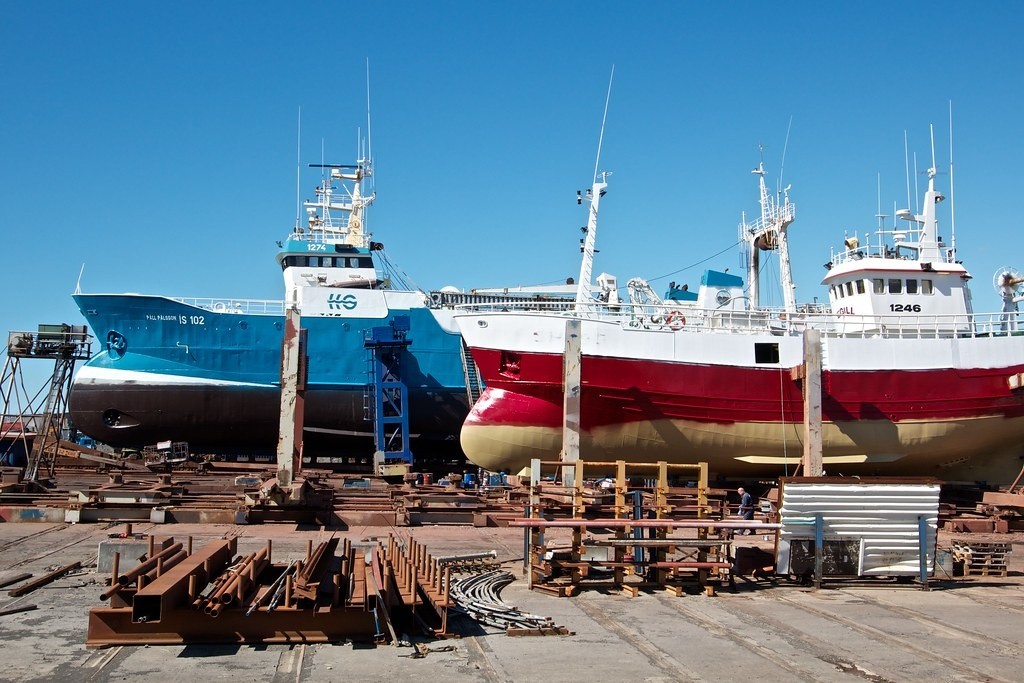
left=667, top=311, right=686, bottom=330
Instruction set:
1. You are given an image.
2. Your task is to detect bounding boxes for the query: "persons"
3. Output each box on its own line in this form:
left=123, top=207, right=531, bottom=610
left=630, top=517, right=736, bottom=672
left=734, top=487, right=757, bottom=536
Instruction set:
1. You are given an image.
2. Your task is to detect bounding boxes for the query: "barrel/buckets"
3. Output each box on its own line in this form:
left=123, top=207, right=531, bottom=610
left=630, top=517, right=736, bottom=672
left=464, top=474, right=475, bottom=488
left=423, top=473, right=433, bottom=484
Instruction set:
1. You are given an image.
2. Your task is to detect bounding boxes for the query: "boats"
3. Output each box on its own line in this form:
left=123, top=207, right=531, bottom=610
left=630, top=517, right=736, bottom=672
left=66, top=54, right=852, bottom=464
left=451, top=63, right=1024, bottom=484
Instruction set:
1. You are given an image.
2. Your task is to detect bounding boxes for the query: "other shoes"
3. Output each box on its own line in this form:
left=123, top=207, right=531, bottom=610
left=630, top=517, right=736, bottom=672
left=748, top=532, right=756, bottom=535
left=735, top=532, right=743, bottom=536
